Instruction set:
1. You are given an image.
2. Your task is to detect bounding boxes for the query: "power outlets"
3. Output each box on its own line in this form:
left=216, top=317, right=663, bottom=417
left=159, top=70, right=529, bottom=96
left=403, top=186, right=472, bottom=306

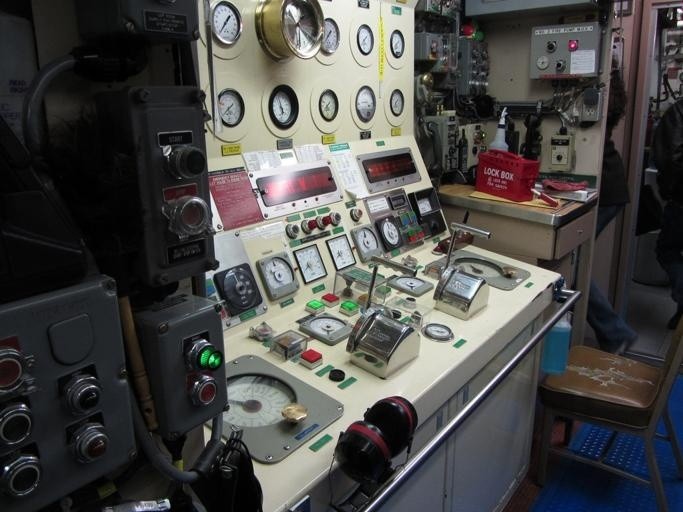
left=577, top=90, right=602, bottom=123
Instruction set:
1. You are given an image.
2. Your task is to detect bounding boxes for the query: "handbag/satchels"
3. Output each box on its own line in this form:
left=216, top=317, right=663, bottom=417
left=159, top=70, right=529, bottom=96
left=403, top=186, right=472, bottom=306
left=635, top=184, right=662, bottom=237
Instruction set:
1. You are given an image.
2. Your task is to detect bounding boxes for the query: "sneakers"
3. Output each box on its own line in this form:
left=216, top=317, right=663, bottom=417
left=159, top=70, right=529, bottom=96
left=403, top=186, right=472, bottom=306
left=597, top=334, right=637, bottom=356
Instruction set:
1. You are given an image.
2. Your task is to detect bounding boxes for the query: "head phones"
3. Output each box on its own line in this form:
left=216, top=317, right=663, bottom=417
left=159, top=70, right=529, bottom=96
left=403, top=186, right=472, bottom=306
left=330, top=395, right=418, bottom=512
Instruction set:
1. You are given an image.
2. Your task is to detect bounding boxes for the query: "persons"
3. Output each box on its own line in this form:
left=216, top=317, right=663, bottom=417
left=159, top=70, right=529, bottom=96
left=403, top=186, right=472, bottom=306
left=651, top=97, right=682, bottom=330
left=567, top=56, right=638, bottom=357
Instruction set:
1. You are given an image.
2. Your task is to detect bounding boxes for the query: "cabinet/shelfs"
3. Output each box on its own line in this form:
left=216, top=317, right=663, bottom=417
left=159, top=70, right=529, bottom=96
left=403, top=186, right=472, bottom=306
left=433, top=181, right=600, bottom=389
left=306, top=315, right=541, bottom=512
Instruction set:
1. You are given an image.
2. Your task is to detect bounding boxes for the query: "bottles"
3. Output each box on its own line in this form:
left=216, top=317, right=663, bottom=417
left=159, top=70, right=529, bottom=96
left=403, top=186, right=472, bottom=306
left=489, top=117, right=510, bottom=151
left=540, top=296, right=572, bottom=375
left=457, top=129, right=469, bottom=172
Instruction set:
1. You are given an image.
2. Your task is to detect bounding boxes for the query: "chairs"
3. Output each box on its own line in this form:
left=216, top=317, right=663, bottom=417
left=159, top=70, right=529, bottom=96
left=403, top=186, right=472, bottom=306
left=533, top=309, right=682, bottom=510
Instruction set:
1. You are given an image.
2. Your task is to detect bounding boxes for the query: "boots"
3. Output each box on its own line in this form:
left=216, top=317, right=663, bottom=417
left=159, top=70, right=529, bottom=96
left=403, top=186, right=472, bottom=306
left=668, top=303, right=683, bottom=330
